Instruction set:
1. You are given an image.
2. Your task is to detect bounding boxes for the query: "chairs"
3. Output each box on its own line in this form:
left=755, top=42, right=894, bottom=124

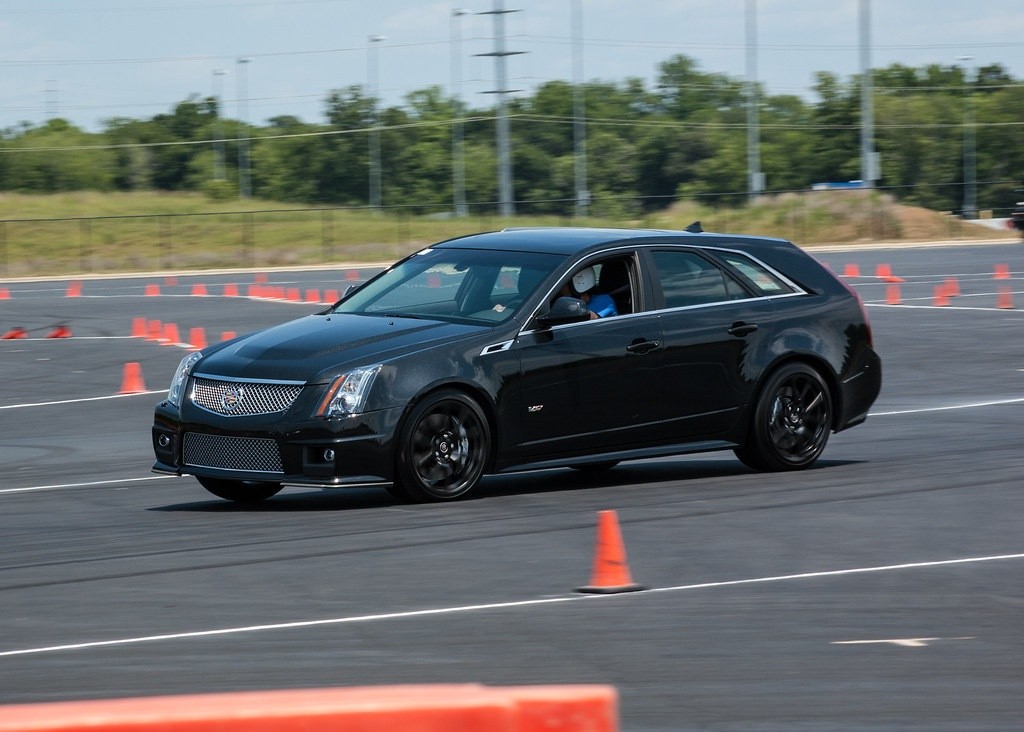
left=599, top=261, right=632, bottom=319
left=501, top=264, right=546, bottom=309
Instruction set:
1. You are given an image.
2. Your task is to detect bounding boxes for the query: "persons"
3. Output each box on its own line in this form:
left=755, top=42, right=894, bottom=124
left=571, top=269, right=618, bottom=320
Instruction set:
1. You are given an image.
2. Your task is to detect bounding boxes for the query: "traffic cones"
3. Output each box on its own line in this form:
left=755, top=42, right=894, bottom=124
left=844, top=256, right=1014, bottom=310
left=0, top=272, right=342, bottom=352
left=113, top=361, right=149, bottom=393
left=573, top=508, right=652, bottom=595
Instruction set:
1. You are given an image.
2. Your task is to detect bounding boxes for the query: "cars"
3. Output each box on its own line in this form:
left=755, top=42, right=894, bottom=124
left=151, top=222, right=881, bottom=505
